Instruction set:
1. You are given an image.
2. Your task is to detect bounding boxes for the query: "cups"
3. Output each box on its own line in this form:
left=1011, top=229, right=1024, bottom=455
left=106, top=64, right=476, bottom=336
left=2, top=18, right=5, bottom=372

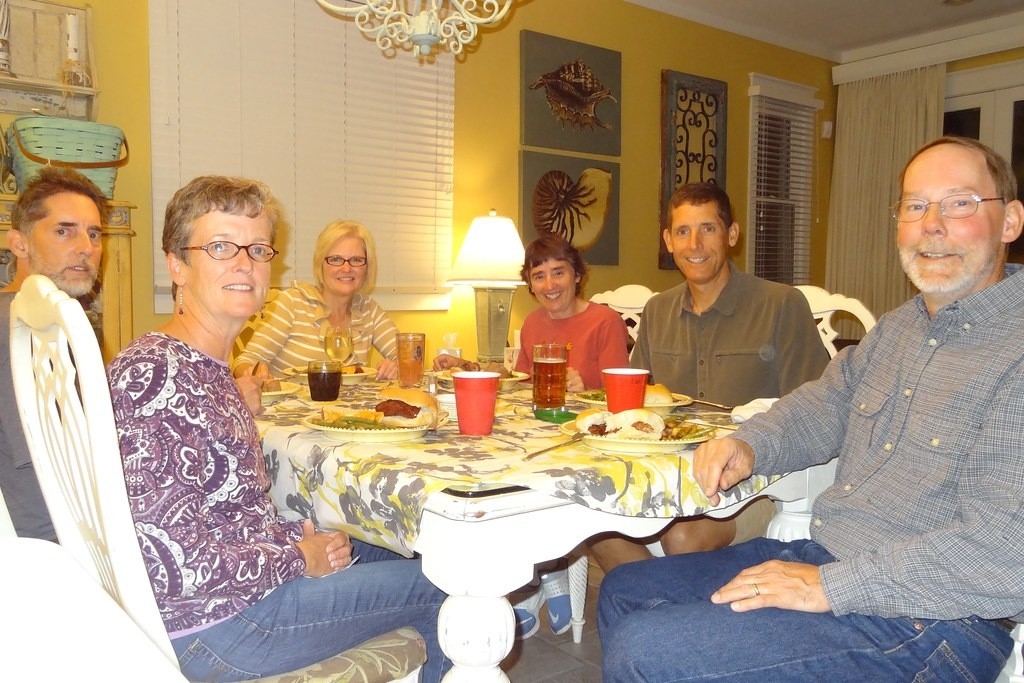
left=602, top=368, right=650, bottom=414
left=533, top=344, right=566, bottom=411
left=308, top=361, right=340, bottom=401
left=451, top=372, right=502, bottom=435
left=396, top=333, right=425, bottom=389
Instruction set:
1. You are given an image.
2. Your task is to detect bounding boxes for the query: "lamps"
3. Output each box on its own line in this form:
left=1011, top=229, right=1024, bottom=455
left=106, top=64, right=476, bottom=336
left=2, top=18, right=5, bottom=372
left=443, top=208, right=526, bottom=363
left=314, top=0, right=514, bottom=62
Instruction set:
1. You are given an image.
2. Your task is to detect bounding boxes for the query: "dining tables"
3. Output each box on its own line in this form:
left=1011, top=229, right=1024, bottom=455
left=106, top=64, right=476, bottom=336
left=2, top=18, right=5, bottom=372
left=251, top=369, right=838, bottom=683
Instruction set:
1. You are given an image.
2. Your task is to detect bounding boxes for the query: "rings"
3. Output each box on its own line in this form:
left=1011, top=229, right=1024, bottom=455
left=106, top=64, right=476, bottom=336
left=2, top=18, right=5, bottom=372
left=752, top=584, right=760, bottom=596
left=568, top=381, right=572, bottom=387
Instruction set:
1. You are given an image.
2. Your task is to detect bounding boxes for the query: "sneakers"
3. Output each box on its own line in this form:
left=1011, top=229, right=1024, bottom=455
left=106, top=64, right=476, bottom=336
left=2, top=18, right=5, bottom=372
left=508, top=574, right=546, bottom=641
left=538, top=557, right=572, bottom=636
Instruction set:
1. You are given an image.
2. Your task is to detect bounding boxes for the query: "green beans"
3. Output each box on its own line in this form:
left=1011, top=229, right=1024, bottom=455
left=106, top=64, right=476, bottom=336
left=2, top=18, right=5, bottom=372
left=664, top=415, right=719, bottom=439
left=318, top=416, right=396, bottom=429
left=577, top=389, right=606, bottom=402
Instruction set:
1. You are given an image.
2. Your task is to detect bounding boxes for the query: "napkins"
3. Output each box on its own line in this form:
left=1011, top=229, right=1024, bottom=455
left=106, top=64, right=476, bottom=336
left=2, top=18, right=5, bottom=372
left=743, top=397, right=780, bottom=424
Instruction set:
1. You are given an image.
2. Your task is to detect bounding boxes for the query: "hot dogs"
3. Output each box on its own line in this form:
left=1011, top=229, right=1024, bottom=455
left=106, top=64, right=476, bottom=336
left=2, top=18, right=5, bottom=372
left=377, top=387, right=439, bottom=428
left=575, top=408, right=665, bottom=441
left=644, top=383, right=674, bottom=406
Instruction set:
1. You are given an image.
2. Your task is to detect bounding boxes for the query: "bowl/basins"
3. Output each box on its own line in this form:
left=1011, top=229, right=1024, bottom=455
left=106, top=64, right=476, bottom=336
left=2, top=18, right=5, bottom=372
left=436, top=394, right=458, bottom=421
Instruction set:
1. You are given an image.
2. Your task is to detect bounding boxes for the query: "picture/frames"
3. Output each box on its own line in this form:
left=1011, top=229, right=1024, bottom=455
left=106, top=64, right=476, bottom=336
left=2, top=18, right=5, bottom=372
left=658, top=69, right=727, bottom=270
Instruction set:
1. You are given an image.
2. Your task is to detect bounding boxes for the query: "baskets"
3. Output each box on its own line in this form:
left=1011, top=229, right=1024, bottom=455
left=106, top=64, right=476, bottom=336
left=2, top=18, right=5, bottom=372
left=4, top=116, right=130, bottom=200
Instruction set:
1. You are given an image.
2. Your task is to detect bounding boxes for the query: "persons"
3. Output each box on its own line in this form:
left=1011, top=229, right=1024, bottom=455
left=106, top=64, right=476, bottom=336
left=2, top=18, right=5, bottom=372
left=596, top=136, right=1023, bottom=683
left=433, top=233, right=630, bottom=640
left=0, top=168, right=106, bottom=545
left=231, top=220, right=401, bottom=381
left=107, top=174, right=454, bottom=683
left=584, top=182, right=833, bottom=575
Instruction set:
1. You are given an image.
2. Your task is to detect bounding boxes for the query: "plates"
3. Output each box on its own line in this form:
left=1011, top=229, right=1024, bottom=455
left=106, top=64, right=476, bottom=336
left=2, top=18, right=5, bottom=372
left=283, top=366, right=377, bottom=384
left=422, top=371, right=530, bottom=390
left=535, top=409, right=577, bottom=423
left=558, top=420, right=715, bottom=453
left=261, top=381, right=300, bottom=406
left=572, top=388, right=694, bottom=414
left=301, top=409, right=427, bottom=441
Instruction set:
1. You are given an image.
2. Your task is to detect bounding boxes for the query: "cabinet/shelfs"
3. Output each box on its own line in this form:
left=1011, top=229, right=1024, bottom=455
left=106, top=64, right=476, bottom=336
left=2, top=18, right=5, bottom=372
left=0, top=194, right=138, bottom=369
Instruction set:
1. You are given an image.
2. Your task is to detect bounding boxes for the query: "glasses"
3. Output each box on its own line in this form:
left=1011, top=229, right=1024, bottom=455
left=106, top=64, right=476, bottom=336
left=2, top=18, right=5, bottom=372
left=323, top=256, right=367, bottom=267
left=889, top=196, right=1004, bottom=223
left=176, top=240, right=280, bottom=263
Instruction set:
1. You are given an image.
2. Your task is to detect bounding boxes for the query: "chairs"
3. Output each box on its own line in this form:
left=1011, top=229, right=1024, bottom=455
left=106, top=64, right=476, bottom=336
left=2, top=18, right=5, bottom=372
left=792, top=284, right=877, bottom=360
left=9, top=273, right=426, bottom=683
left=588, top=284, right=661, bottom=362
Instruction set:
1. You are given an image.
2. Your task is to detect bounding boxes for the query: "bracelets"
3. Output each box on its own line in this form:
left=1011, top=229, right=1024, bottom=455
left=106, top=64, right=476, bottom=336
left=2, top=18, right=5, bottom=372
left=473, top=362, right=480, bottom=371
